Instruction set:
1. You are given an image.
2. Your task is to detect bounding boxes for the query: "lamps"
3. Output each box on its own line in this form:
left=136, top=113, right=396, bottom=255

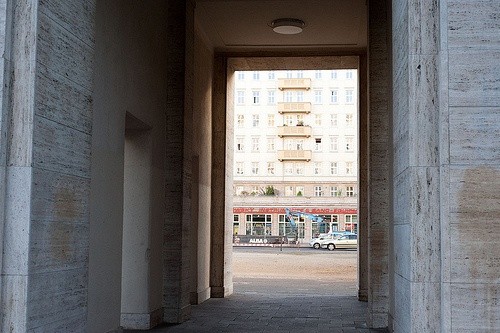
left=270, top=18, right=306, bottom=36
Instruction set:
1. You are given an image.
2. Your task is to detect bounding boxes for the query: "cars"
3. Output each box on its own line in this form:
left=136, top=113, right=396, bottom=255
left=311, top=233, right=343, bottom=249
left=322, top=234, right=358, bottom=250
